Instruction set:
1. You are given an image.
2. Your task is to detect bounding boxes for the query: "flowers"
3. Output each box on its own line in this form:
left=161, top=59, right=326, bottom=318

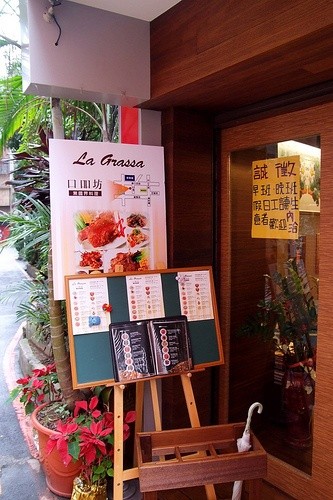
left=45, top=394, right=137, bottom=486
left=7, top=362, right=62, bottom=413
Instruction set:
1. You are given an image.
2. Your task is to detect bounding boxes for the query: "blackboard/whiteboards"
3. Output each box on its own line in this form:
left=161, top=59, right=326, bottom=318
left=63, top=266, right=224, bottom=391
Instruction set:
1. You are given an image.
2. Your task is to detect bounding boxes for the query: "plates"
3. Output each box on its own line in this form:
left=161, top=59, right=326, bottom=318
left=79, top=216, right=149, bottom=249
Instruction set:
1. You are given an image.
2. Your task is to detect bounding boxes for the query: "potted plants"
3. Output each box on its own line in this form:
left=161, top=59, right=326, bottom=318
left=1, top=191, right=111, bottom=498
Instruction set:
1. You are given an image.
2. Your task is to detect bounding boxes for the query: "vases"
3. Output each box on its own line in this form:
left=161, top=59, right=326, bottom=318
left=69, top=476, right=108, bottom=500
